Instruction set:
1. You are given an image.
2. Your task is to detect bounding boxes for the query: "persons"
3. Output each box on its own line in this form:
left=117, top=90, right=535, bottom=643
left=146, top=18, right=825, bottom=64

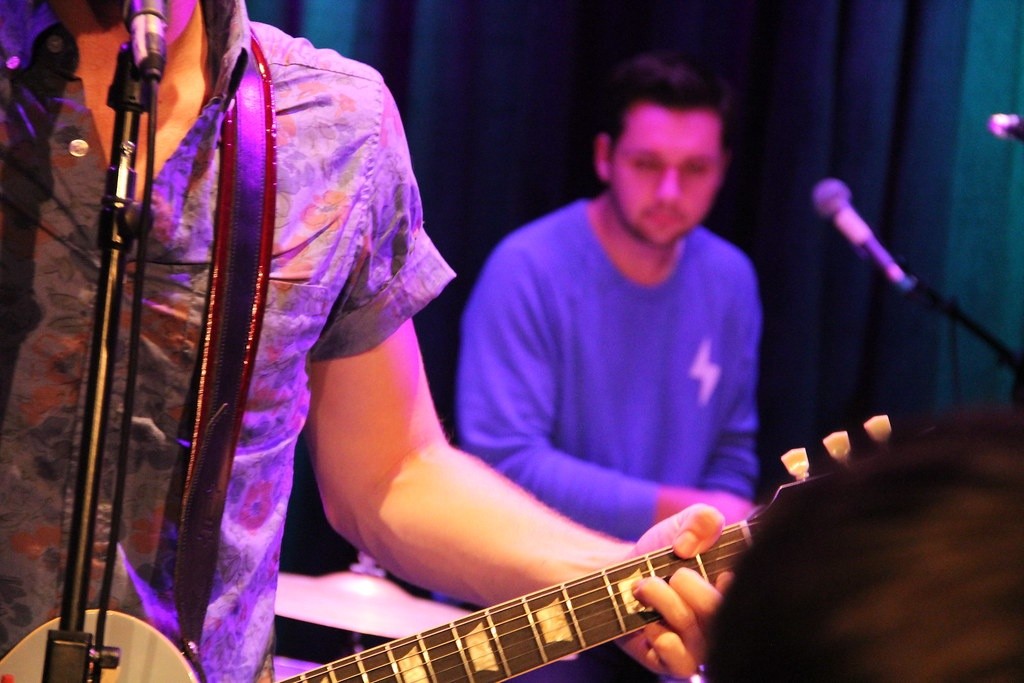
left=0, top=0, right=734, bottom=683
left=430, top=42, right=761, bottom=683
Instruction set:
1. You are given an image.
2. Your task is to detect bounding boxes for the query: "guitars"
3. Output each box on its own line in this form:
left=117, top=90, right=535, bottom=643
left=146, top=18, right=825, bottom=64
left=0, top=401, right=905, bottom=683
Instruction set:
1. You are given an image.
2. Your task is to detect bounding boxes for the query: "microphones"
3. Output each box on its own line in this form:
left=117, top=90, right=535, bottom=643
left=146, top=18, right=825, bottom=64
left=812, top=179, right=916, bottom=293
left=120, top=0, right=169, bottom=83
left=989, top=113, right=1024, bottom=143
